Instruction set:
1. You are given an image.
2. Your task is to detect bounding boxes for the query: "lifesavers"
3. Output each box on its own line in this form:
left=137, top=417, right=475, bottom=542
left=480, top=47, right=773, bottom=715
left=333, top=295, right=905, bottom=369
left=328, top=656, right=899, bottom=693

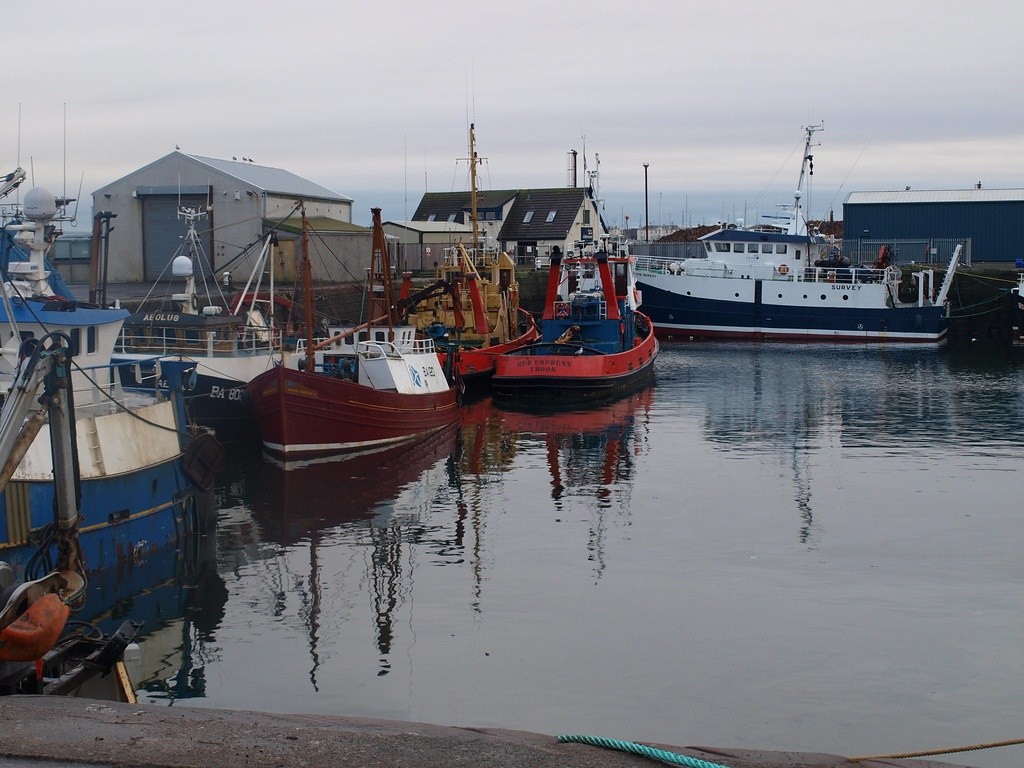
left=779, top=264, right=789, bottom=276
left=633, top=288, right=638, bottom=303
left=556, top=304, right=570, bottom=317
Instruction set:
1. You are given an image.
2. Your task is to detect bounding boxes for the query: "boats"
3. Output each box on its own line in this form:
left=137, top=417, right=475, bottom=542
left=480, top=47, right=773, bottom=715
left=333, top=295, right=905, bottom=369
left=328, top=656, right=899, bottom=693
left=107, top=205, right=327, bottom=431
left=245, top=204, right=460, bottom=477
left=0, top=187, right=216, bottom=699
left=492, top=167, right=661, bottom=412
left=410, top=120, right=540, bottom=406
left=635, top=190, right=963, bottom=350
